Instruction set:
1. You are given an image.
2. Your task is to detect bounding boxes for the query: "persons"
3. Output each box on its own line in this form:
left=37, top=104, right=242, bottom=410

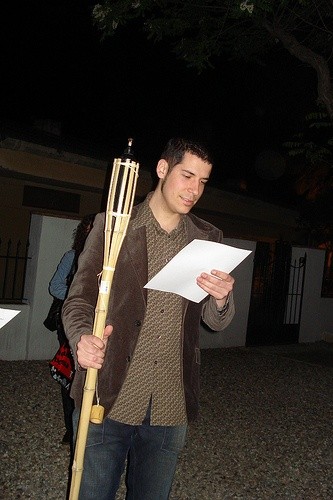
left=63, top=130, right=236, bottom=500
left=45, top=212, right=98, bottom=444
